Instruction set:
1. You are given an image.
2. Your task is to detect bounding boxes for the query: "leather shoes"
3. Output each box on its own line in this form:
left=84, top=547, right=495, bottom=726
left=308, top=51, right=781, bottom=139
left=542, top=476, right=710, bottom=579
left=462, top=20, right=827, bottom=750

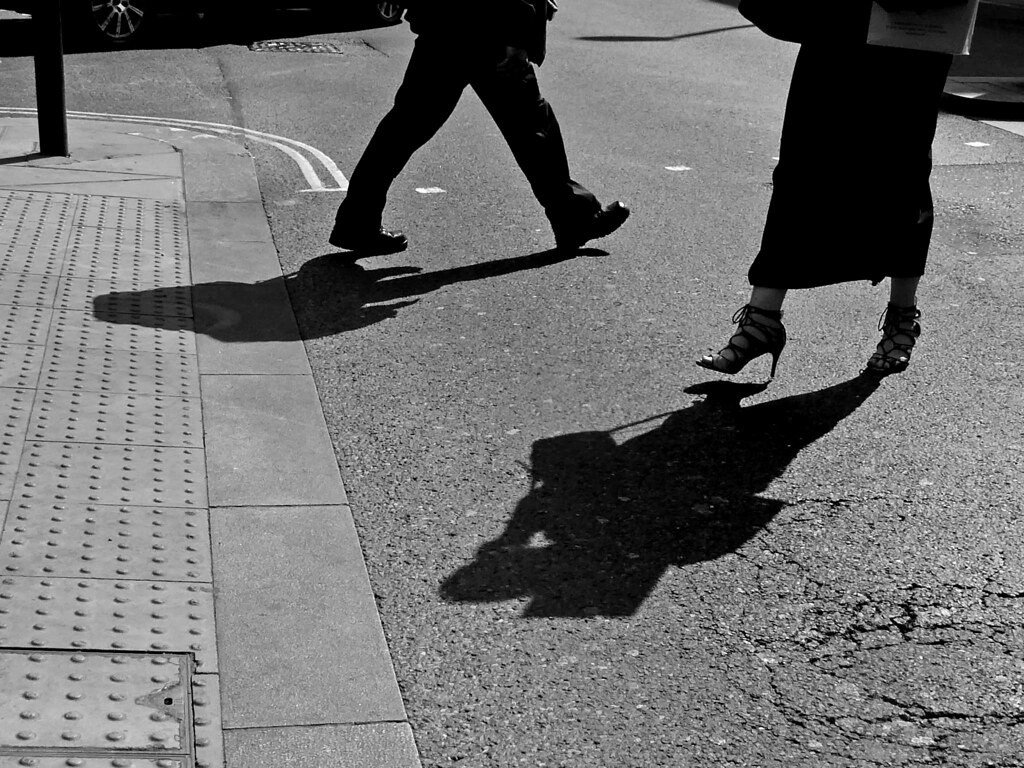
left=556, top=201, right=629, bottom=250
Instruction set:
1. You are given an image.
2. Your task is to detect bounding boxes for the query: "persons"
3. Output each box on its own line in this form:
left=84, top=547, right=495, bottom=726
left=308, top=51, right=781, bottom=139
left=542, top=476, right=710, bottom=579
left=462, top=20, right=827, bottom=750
left=696, top=0, right=961, bottom=375
left=329, top=0, right=630, bottom=254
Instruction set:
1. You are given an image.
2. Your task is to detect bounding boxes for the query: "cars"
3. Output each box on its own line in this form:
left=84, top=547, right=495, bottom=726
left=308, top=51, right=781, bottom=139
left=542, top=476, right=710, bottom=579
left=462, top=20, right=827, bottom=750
left=1, top=0, right=413, bottom=51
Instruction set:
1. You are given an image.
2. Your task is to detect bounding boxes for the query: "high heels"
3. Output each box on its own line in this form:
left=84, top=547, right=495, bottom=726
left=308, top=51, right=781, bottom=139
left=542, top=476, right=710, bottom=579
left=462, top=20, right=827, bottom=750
left=696, top=304, right=787, bottom=378
left=328, top=223, right=408, bottom=253
left=868, top=301, right=922, bottom=370
